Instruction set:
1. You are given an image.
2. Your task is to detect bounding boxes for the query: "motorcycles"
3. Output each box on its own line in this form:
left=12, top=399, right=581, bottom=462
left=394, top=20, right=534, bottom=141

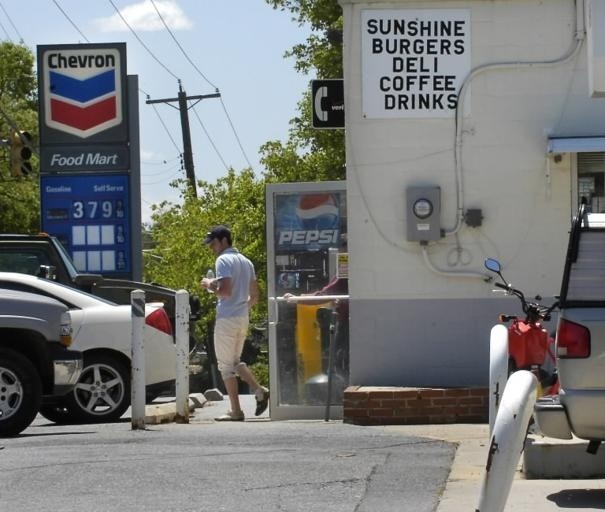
left=484, top=258, right=560, bottom=424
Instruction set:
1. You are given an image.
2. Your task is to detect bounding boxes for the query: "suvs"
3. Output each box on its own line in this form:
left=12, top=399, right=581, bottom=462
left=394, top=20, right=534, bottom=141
left=0, top=233, right=200, bottom=404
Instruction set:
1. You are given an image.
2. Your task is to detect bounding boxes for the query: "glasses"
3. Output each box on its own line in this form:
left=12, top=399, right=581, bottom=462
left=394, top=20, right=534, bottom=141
left=207, top=231, right=217, bottom=237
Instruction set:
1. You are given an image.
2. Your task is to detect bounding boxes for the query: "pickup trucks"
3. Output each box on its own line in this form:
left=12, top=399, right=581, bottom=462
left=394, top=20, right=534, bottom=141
left=533, top=299, right=605, bottom=454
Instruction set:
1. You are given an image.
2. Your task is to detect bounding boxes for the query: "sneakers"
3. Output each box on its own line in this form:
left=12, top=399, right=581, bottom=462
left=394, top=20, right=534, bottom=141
left=214, top=410, right=244, bottom=421
left=255, top=387, right=269, bottom=416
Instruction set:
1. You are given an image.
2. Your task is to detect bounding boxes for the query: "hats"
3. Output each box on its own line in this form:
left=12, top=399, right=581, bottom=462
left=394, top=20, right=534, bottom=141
left=204, top=225, right=231, bottom=244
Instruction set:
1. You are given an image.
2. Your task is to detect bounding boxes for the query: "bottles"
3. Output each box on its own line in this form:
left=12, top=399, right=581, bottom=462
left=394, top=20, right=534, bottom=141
left=206, top=269, right=215, bottom=293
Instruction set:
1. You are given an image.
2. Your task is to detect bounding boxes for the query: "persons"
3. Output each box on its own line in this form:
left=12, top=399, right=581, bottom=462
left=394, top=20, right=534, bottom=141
left=283, top=233, right=348, bottom=378
left=200, top=226, right=270, bottom=422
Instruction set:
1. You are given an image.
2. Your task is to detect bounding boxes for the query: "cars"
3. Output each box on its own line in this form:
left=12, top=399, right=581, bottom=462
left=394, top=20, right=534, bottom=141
left=0, top=272, right=181, bottom=425
left=0, top=288, right=74, bottom=441
